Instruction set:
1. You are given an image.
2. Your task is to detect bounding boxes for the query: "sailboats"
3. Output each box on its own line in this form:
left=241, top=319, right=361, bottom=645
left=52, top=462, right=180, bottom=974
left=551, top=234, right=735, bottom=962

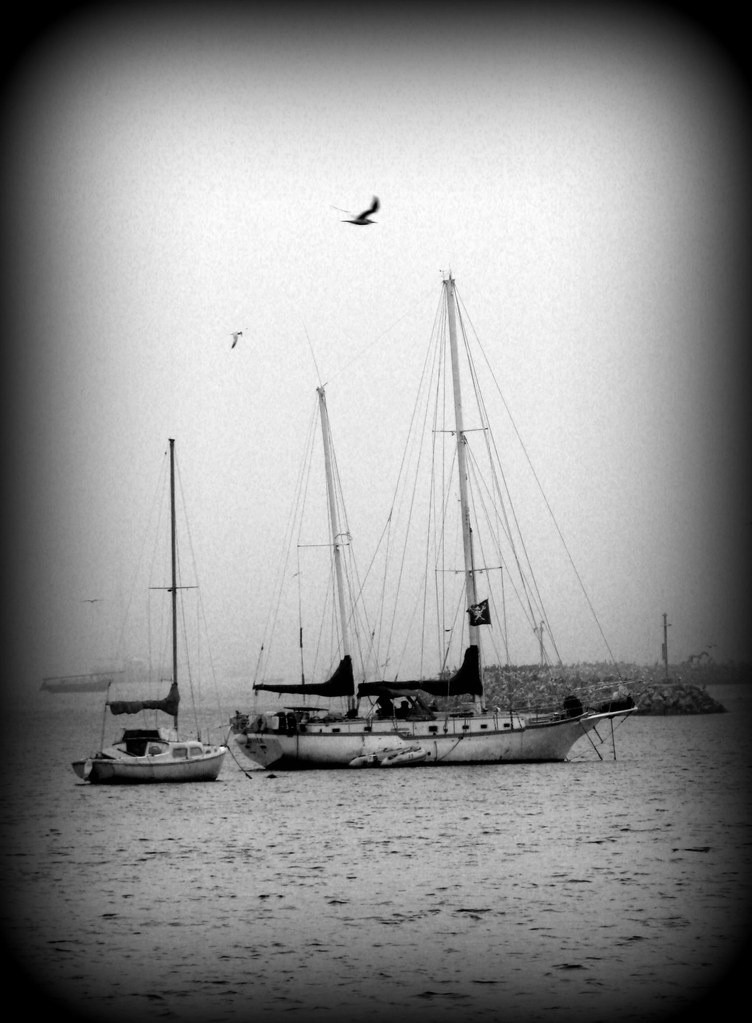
left=224, top=267, right=644, bottom=769
left=68, top=436, right=230, bottom=780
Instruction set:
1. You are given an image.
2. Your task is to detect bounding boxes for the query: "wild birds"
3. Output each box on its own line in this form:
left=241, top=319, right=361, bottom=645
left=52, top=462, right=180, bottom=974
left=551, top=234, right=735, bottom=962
left=230, top=331, right=242, bottom=348
left=342, top=195, right=379, bottom=225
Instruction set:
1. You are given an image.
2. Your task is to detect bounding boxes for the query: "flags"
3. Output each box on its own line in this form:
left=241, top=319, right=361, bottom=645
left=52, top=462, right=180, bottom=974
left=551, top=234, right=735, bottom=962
left=467, top=599, right=492, bottom=626
